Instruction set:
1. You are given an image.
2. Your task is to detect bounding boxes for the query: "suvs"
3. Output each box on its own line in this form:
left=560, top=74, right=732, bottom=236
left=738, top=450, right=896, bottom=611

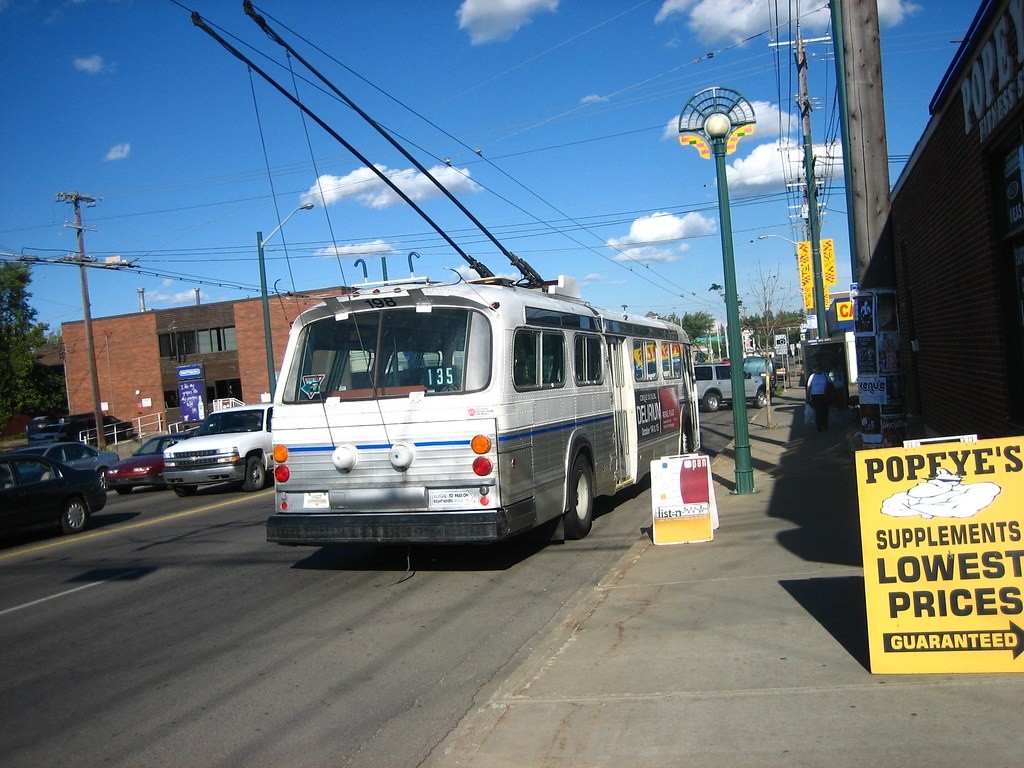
left=691, top=362, right=771, bottom=412
left=160, top=402, right=274, bottom=497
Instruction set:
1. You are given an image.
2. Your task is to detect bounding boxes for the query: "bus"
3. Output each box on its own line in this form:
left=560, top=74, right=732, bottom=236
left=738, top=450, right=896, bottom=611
left=186, top=2, right=703, bottom=548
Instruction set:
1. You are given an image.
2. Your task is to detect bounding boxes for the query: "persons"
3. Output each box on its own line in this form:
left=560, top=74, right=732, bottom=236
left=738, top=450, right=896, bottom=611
left=861, top=301, right=872, bottom=328
left=805, top=361, right=836, bottom=433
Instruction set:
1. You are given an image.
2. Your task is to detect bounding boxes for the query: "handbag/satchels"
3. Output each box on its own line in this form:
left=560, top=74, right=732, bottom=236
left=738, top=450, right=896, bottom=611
left=824, top=372, right=838, bottom=402
left=804, top=402, right=816, bottom=424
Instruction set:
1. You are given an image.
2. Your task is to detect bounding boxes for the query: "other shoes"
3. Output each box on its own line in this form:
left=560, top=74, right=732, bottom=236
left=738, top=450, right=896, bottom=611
left=817, top=427, right=822, bottom=432
left=824, top=426, right=829, bottom=429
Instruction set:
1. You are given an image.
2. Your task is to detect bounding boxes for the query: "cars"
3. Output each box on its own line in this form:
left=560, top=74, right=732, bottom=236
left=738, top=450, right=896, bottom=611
left=27, top=411, right=133, bottom=449
left=0, top=454, right=108, bottom=542
left=104, top=431, right=194, bottom=495
left=8, top=441, right=121, bottom=492
left=741, top=357, right=778, bottom=389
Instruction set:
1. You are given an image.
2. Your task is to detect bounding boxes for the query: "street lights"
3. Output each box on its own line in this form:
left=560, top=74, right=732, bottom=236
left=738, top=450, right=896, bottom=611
left=254, top=202, right=315, bottom=401
left=677, top=86, right=758, bottom=496
left=758, top=235, right=814, bottom=340
